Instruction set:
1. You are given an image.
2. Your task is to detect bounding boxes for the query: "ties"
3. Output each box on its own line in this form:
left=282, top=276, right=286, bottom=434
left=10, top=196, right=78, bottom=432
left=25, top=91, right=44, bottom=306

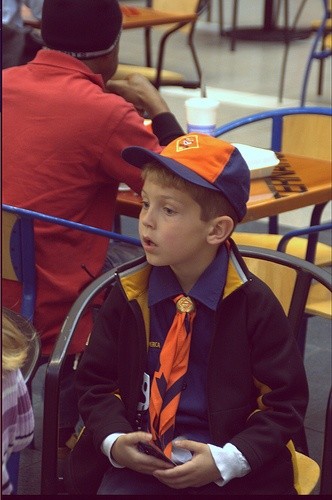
left=147, top=293, right=199, bottom=463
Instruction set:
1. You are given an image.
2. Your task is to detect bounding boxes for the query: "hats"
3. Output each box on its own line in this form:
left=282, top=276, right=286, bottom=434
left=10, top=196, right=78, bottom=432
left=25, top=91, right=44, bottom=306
left=41, top=0, right=123, bottom=60
left=120, top=132, right=251, bottom=224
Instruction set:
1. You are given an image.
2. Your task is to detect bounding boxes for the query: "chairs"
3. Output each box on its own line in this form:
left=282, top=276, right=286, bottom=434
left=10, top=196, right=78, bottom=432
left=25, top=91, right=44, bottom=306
left=0, top=0, right=332, bottom=500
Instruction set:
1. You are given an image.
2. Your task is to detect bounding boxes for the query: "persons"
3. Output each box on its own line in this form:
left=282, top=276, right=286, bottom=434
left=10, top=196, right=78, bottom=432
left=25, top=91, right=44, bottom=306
left=0, top=0, right=43, bottom=68
left=74, top=134, right=310, bottom=495
left=1, top=307, right=36, bottom=496
left=2, top=0, right=188, bottom=360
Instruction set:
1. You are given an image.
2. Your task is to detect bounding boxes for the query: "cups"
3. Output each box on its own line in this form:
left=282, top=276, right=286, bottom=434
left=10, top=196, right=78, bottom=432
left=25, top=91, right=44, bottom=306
left=184, top=97, right=220, bottom=138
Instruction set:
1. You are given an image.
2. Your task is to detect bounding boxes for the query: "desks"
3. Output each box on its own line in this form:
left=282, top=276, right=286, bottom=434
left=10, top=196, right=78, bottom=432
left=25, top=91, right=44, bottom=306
left=114, top=148, right=332, bottom=264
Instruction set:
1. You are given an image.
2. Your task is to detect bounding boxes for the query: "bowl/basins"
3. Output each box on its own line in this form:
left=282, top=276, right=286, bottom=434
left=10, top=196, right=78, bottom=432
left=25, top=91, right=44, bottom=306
left=232, top=143, right=280, bottom=178
